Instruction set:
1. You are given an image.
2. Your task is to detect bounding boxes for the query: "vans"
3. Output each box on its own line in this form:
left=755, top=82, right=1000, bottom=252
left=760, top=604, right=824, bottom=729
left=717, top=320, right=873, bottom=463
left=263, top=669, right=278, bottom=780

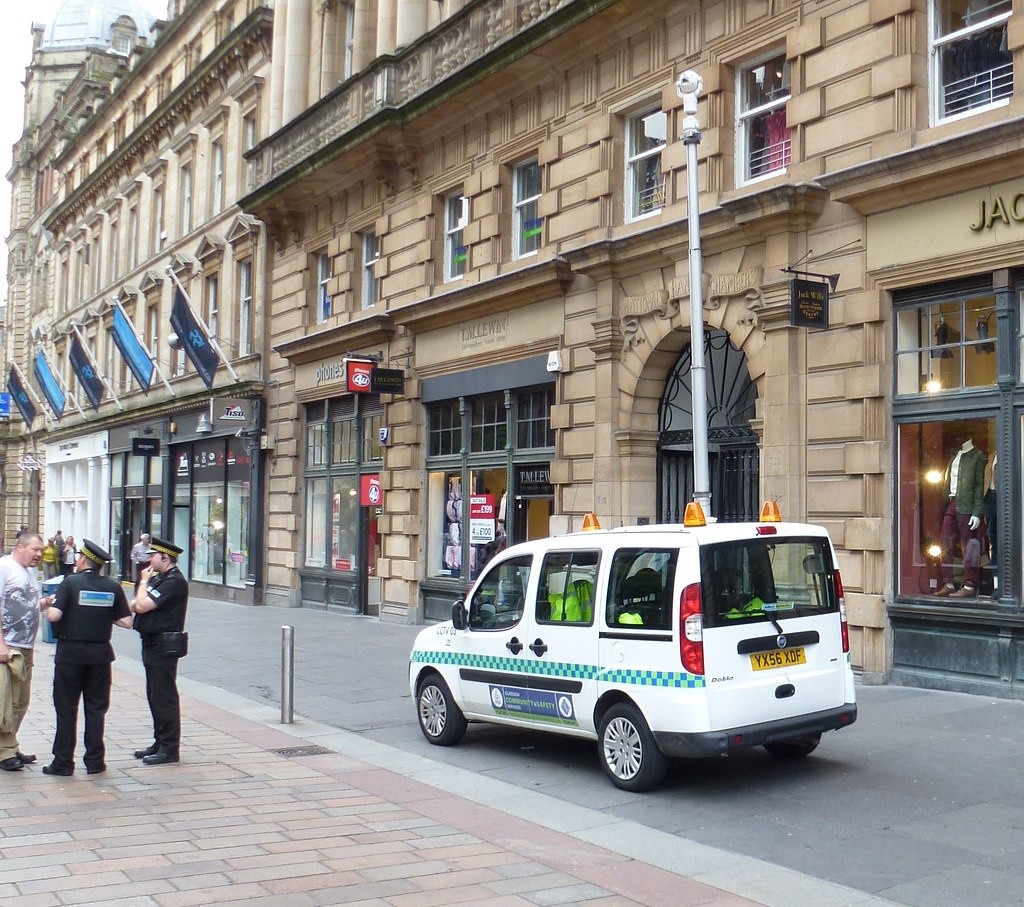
left=406, top=501, right=858, bottom=794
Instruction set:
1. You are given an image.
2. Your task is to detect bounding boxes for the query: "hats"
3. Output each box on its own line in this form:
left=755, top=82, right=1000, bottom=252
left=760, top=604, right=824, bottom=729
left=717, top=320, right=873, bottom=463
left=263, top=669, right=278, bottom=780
left=144, top=536, right=183, bottom=559
left=75, top=539, right=113, bottom=566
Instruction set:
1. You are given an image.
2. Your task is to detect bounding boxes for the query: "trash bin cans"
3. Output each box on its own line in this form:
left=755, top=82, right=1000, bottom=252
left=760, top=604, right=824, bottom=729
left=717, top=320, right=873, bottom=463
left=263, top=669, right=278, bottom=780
left=41, top=575, right=64, bottom=643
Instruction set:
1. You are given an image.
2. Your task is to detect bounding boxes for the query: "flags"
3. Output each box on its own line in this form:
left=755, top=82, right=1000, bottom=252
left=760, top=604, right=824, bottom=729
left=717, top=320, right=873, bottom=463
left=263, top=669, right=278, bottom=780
left=69, top=335, right=104, bottom=413
left=6, top=368, right=36, bottom=429
left=34, top=350, right=67, bottom=421
left=170, top=288, right=219, bottom=393
left=111, top=306, right=154, bottom=396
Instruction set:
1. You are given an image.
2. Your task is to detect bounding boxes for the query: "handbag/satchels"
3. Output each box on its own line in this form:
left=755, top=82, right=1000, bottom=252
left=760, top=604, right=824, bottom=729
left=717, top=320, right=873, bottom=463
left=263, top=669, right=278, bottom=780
left=73, top=566, right=77, bottom=573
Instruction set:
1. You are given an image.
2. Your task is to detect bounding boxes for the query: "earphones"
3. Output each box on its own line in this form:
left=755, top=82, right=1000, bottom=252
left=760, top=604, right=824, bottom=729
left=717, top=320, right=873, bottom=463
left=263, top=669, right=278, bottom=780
left=161, top=556, right=166, bottom=560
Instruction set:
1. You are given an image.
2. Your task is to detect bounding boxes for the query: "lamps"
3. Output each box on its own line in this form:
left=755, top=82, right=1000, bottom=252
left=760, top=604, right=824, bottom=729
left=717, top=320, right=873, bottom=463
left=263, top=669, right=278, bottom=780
left=975, top=315, right=995, bottom=355
left=931, top=316, right=955, bottom=359
left=196, top=420, right=212, bottom=433
left=168, top=332, right=186, bottom=351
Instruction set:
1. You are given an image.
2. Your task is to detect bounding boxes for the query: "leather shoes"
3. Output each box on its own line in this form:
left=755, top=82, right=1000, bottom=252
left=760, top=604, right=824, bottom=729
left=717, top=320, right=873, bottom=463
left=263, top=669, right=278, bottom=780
left=142, top=751, right=179, bottom=763
left=0, top=757, right=24, bottom=769
left=934, top=585, right=955, bottom=596
left=948, top=587, right=976, bottom=598
left=42, top=763, right=74, bottom=776
left=134, top=748, right=156, bottom=758
left=16, top=751, right=36, bottom=762
left=87, top=763, right=106, bottom=773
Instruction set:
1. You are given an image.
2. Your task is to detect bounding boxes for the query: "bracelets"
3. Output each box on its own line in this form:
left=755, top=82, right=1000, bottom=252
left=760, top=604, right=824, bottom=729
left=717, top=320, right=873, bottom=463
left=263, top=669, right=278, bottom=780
left=140, top=580, right=147, bottom=584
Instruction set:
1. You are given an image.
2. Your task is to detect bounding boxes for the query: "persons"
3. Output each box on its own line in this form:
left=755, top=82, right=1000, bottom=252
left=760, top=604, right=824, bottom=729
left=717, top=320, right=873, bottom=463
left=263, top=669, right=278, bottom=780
left=16, top=527, right=28, bottom=538
left=0, top=532, right=52, bottom=770
left=43, top=530, right=77, bottom=580
left=43, top=538, right=133, bottom=775
left=131, top=534, right=151, bottom=596
left=935, top=439, right=997, bottom=597
left=131, top=537, right=189, bottom=763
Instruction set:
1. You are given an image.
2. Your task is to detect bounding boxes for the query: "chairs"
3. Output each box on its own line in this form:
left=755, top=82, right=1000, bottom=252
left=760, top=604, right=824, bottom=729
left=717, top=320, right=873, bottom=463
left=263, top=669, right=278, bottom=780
left=549, top=580, right=594, bottom=622
left=621, top=568, right=749, bottom=628
